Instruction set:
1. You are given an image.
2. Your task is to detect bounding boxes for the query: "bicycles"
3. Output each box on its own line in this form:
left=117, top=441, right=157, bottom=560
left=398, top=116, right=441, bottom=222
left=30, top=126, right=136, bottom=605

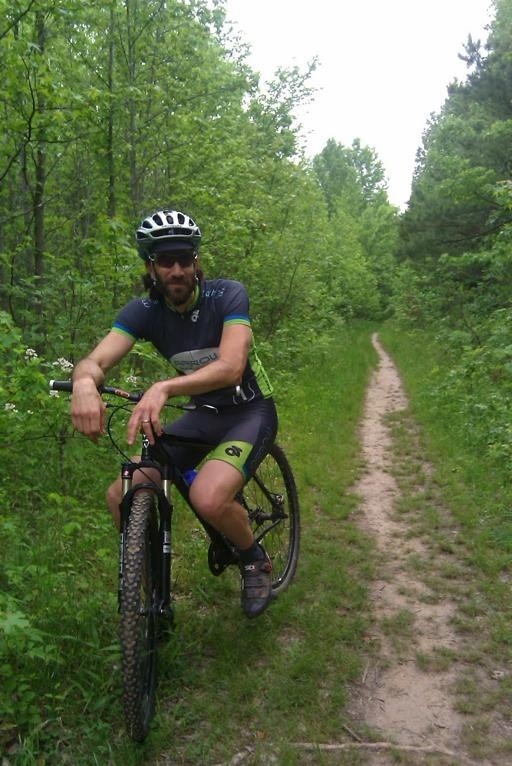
left=47, top=379, right=302, bottom=746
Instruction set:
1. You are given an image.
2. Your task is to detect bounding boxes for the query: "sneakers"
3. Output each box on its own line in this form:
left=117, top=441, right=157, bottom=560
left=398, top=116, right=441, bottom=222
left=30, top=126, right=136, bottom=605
left=240, top=544, right=272, bottom=618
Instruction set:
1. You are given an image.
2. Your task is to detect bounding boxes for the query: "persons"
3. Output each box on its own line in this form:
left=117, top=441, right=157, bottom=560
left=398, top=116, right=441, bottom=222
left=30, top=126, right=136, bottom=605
left=69, top=208, right=281, bottom=645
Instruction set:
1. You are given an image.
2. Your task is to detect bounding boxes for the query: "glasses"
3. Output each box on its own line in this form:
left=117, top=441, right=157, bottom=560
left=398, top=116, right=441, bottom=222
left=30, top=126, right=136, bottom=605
left=156, top=254, right=194, bottom=268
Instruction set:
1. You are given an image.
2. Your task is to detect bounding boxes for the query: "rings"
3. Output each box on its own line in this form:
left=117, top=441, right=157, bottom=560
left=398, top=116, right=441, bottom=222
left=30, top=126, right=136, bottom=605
left=140, top=420, right=150, bottom=423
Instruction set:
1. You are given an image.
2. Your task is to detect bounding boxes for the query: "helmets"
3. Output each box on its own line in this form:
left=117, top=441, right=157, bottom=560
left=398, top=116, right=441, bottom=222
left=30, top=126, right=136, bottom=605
left=137, top=210, right=201, bottom=259
